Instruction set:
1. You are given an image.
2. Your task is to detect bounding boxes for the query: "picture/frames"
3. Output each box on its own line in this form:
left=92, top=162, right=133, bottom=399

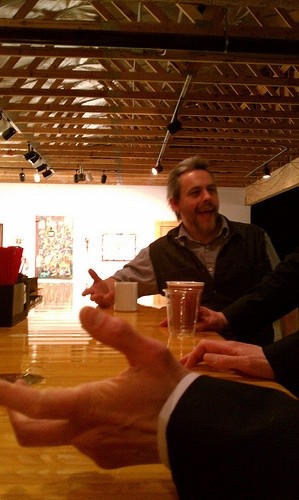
left=102, top=233, right=137, bottom=262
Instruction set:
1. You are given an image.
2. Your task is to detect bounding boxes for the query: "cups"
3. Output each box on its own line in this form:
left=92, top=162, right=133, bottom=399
left=167, top=336, right=195, bottom=360
left=114, top=281, right=138, bottom=312
left=162, top=281, right=205, bottom=338
left=0, top=246, right=24, bottom=284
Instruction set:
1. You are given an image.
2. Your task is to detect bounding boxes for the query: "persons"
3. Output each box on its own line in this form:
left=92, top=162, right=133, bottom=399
left=0, top=306, right=299, bottom=500
left=160, top=248, right=299, bottom=382
left=82, top=155, right=299, bottom=346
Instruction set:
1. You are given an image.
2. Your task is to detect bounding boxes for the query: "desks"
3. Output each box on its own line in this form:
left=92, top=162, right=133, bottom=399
left=0, top=309, right=295, bottom=500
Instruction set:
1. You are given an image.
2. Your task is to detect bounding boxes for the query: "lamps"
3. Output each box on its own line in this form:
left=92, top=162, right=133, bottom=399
left=262, top=168, right=271, bottom=179
left=1, top=127, right=16, bottom=141
left=71, top=170, right=93, bottom=183
left=100, top=172, right=108, bottom=185
left=152, top=165, right=163, bottom=176
left=19, top=144, right=53, bottom=183
left=167, top=121, right=183, bottom=134
